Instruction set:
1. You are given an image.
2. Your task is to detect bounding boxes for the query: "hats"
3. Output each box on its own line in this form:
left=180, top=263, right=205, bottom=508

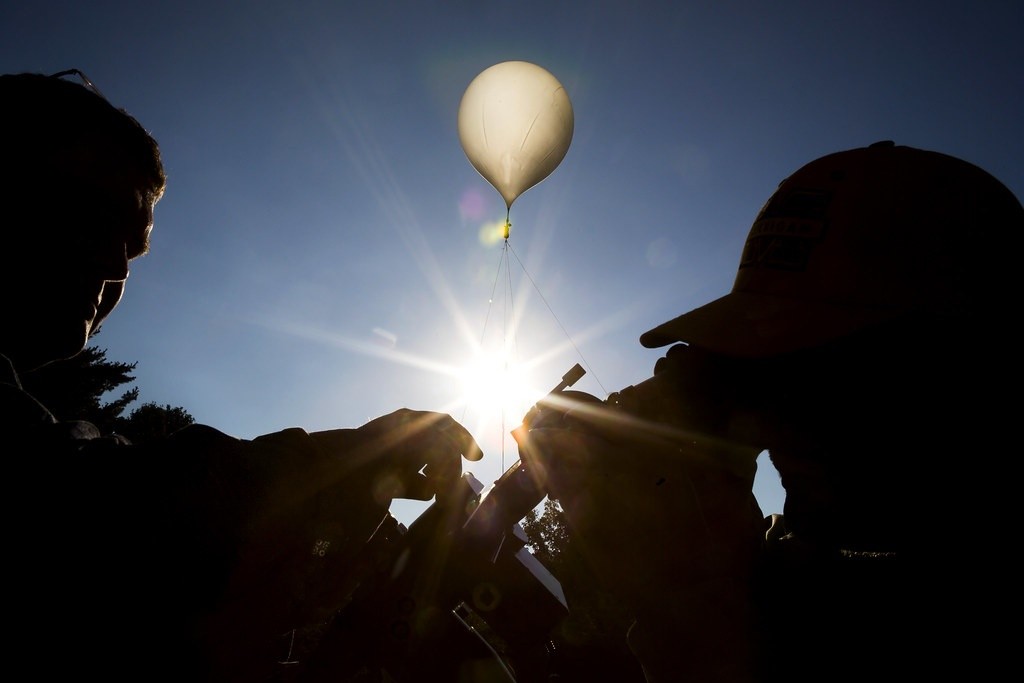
left=640, top=140, right=1024, bottom=352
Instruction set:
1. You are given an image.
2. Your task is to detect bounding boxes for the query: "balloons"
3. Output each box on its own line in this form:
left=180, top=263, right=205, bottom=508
left=459, top=61, right=574, bottom=241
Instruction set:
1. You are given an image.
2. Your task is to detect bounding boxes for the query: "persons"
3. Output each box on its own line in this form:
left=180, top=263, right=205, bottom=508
left=0, top=71, right=1023, bottom=683
